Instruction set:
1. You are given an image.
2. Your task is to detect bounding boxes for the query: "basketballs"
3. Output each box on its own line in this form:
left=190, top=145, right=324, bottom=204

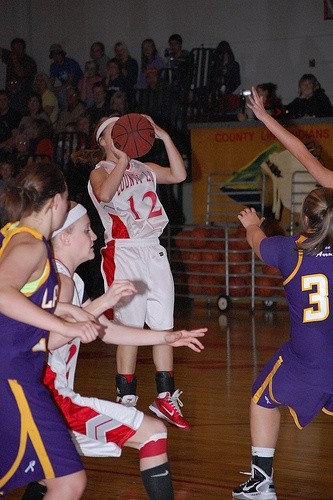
left=111, top=113, right=155, bottom=158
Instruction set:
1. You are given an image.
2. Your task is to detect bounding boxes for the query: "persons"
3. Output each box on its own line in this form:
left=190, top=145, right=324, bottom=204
left=0, top=34, right=333, bottom=500
left=232, top=187, right=333, bottom=500
left=88, top=113, right=193, bottom=430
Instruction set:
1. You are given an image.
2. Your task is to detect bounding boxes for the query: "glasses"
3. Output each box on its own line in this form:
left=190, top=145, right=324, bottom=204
left=17, top=141, right=27, bottom=145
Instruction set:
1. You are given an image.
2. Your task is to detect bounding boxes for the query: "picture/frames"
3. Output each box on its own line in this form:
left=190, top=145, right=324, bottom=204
left=324, top=0, right=333, bottom=20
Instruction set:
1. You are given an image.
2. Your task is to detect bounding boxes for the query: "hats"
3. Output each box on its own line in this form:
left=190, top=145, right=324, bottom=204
left=49, top=44, right=67, bottom=59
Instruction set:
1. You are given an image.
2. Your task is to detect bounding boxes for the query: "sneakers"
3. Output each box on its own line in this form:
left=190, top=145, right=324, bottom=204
left=149, top=390, right=190, bottom=428
left=232, top=464, right=277, bottom=500
left=116, top=394, right=140, bottom=408
